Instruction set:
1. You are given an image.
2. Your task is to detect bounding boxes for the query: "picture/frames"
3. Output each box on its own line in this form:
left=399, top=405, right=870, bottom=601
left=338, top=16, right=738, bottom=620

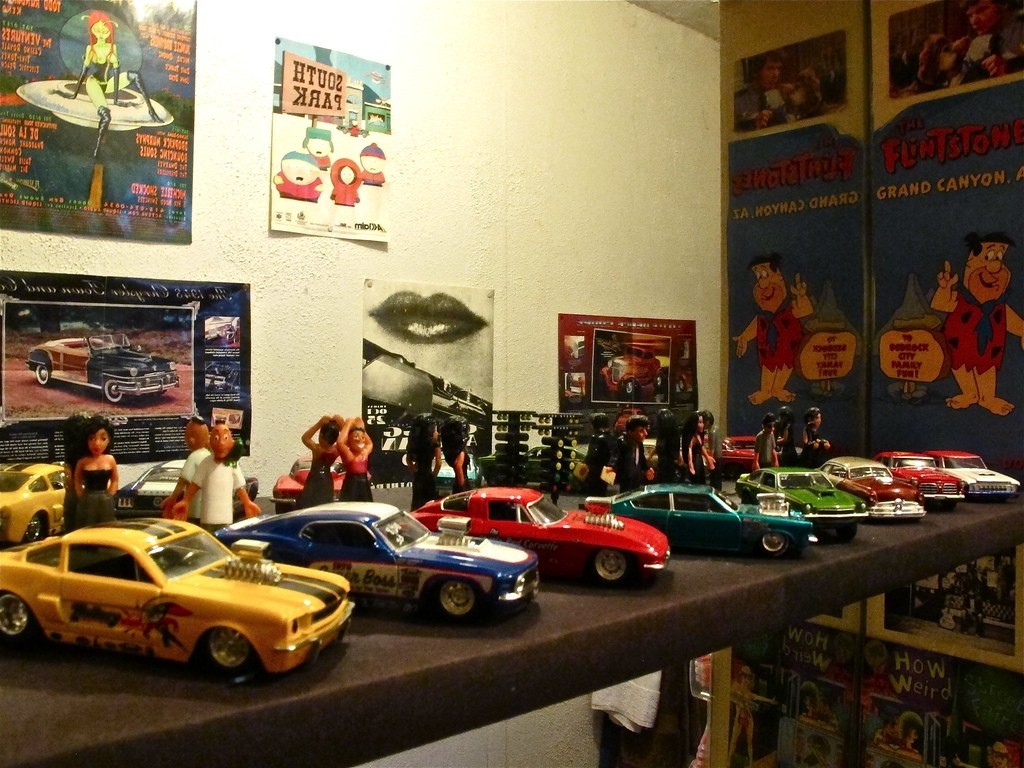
left=804, top=602, right=862, bottom=633
left=866, top=544, right=1024, bottom=674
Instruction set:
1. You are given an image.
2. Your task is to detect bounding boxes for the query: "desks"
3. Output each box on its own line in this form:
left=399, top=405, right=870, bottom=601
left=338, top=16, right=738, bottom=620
left=0, top=482, right=1024, bottom=768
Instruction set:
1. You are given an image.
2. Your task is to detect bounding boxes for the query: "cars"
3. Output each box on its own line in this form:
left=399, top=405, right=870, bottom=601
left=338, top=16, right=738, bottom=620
left=0, top=461, right=68, bottom=544
left=113, top=461, right=259, bottom=520
left=24, top=333, right=180, bottom=405
left=814, top=455, right=927, bottom=520
left=872, top=451, right=967, bottom=510
left=585, top=482, right=813, bottom=559
left=0, top=516, right=357, bottom=678
left=411, top=487, right=671, bottom=592
left=214, top=501, right=539, bottom=627
left=929, top=449, right=1021, bottom=503
left=735, top=466, right=870, bottom=542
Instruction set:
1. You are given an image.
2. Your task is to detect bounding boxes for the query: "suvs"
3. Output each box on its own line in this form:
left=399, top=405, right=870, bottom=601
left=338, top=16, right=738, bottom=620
left=606, top=345, right=667, bottom=400
